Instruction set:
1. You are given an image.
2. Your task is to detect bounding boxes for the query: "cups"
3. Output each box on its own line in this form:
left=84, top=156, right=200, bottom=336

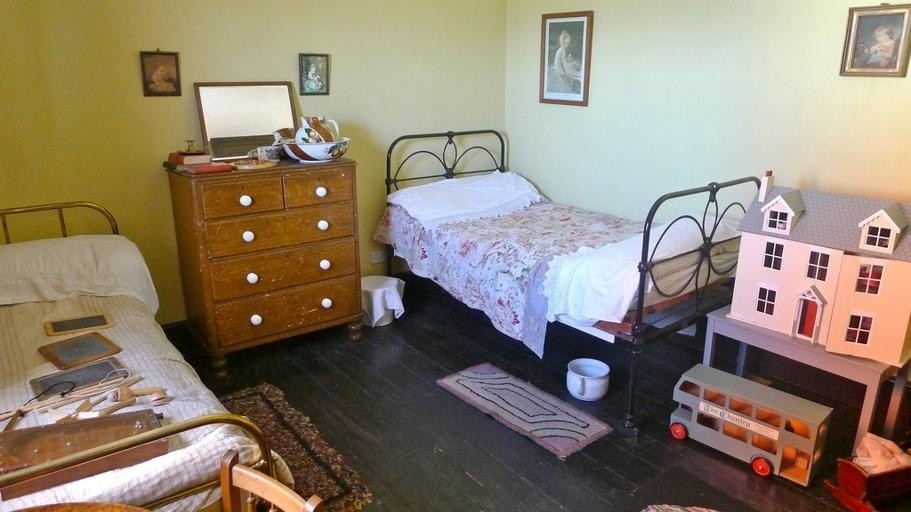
left=257, top=146, right=282, bottom=163
left=567, top=356, right=613, bottom=404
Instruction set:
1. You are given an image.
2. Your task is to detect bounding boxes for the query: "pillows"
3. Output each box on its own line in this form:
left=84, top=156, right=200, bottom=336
left=384, top=169, right=542, bottom=233
left=0, top=234, right=160, bottom=317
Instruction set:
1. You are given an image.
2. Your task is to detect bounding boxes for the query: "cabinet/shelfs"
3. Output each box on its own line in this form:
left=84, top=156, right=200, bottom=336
left=162, top=152, right=364, bottom=378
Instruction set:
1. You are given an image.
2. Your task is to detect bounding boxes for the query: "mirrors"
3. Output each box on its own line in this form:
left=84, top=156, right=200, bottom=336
left=194, top=81, right=298, bottom=154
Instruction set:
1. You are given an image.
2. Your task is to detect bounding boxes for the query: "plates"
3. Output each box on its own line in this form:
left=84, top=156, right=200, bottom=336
left=231, top=161, right=278, bottom=169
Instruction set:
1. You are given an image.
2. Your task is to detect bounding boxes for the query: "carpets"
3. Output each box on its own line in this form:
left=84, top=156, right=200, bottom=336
left=437, top=362, right=613, bottom=461
left=217, top=382, right=373, bottom=512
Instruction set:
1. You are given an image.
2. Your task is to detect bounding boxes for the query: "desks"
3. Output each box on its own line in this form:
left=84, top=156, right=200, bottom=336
left=701, top=301, right=908, bottom=459
left=10, top=501, right=152, bottom=512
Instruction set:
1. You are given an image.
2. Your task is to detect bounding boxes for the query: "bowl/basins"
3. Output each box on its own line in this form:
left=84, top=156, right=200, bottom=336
left=281, top=139, right=352, bottom=162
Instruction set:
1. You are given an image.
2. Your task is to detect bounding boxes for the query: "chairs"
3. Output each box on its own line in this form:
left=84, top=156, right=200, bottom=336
left=220, top=449, right=324, bottom=512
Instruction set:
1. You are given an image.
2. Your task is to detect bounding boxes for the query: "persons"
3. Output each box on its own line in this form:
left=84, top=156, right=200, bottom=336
left=145, top=55, right=176, bottom=92
left=554, top=30, right=575, bottom=93
left=868, top=23, right=896, bottom=68
left=304, top=64, right=327, bottom=93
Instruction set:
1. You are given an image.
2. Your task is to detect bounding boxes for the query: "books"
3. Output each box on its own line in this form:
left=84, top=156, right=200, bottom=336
left=186, top=161, right=231, bottom=174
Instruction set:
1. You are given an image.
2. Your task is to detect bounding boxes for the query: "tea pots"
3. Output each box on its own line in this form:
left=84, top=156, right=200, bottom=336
left=296, top=115, right=342, bottom=143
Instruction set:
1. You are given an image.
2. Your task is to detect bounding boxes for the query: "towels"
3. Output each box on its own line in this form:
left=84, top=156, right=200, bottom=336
left=851, top=432, right=911, bottom=474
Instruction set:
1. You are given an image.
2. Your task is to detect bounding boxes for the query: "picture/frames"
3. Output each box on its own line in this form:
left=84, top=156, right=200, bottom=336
left=45, top=313, right=115, bottom=336
left=839, top=3, right=911, bottom=77
left=38, top=331, right=122, bottom=370
left=140, top=50, right=182, bottom=97
left=539, top=11, right=593, bottom=106
left=29, top=356, right=128, bottom=401
left=299, top=53, right=330, bottom=95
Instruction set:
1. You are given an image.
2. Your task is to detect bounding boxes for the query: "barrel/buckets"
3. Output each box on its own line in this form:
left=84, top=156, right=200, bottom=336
left=361, top=276, right=405, bottom=327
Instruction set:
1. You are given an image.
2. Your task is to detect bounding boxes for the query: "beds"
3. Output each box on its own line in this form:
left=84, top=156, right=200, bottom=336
left=371, top=131, right=762, bottom=429
left=0, top=201, right=297, bottom=512
